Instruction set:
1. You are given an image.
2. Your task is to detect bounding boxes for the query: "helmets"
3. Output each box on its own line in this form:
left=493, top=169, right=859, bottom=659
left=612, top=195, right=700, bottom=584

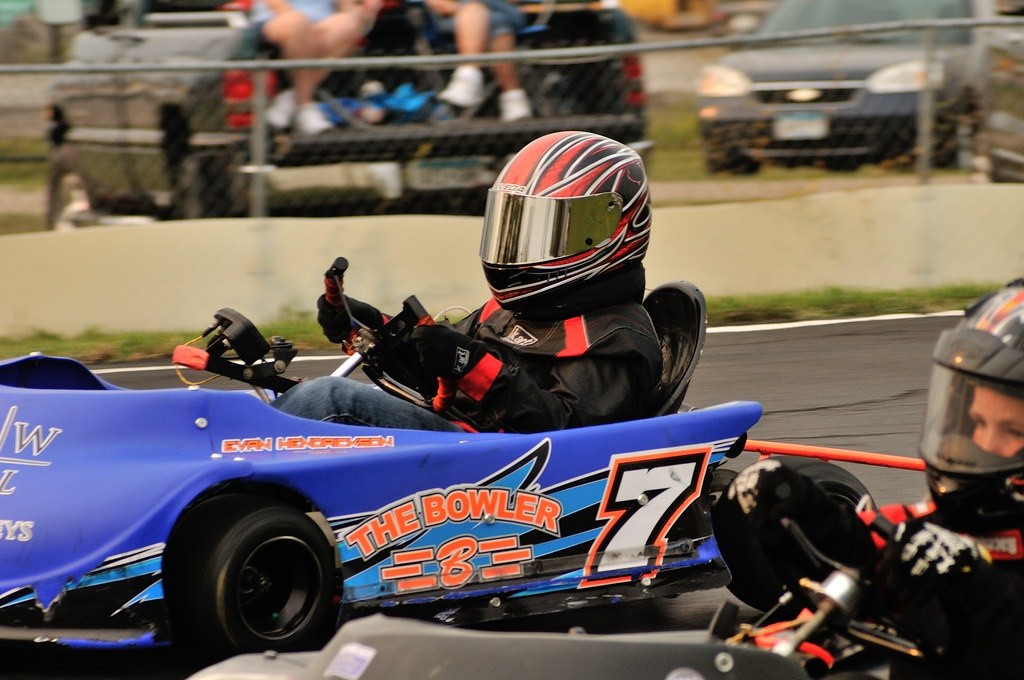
left=918, top=279, right=1024, bottom=532
left=478, top=130, right=652, bottom=326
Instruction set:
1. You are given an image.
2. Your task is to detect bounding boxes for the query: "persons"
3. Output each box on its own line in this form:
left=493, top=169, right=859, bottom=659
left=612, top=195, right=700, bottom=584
left=717, top=276, right=1024, bottom=680
left=268, top=132, right=663, bottom=436
left=426, top=0, right=532, bottom=123
left=248, top=1, right=385, bottom=130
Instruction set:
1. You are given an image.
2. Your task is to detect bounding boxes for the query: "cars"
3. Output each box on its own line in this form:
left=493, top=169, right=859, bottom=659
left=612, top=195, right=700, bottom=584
left=971, top=23, right=1024, bottom=183
left=698, top=0, right=1000, bottom=176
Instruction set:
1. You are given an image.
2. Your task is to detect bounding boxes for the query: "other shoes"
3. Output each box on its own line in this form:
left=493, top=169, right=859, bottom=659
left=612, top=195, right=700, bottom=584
left=360, top=82, right=389, bottom=123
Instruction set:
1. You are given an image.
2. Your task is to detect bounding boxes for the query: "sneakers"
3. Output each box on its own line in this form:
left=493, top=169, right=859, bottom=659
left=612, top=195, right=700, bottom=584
left=497, top=89, right=535, bottom=124
left=267, top=88, right=294, bottom=131
left=434, top=65, right=487, bottom=108
left=294, top=104, right=334, bottom=137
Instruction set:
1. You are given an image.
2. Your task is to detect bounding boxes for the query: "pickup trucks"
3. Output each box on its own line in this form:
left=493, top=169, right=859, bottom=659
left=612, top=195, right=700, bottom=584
left=52, top=2, right=653, bottom=225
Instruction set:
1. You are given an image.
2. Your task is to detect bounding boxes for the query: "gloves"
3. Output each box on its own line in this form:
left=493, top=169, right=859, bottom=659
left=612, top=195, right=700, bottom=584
left=407, top=324, right=489, bottom=379
left=317, top=294, right=385, bottom=344
left=878, top=526, right=986, bottom=637
left=723, top=458, right=817, bottom=554
